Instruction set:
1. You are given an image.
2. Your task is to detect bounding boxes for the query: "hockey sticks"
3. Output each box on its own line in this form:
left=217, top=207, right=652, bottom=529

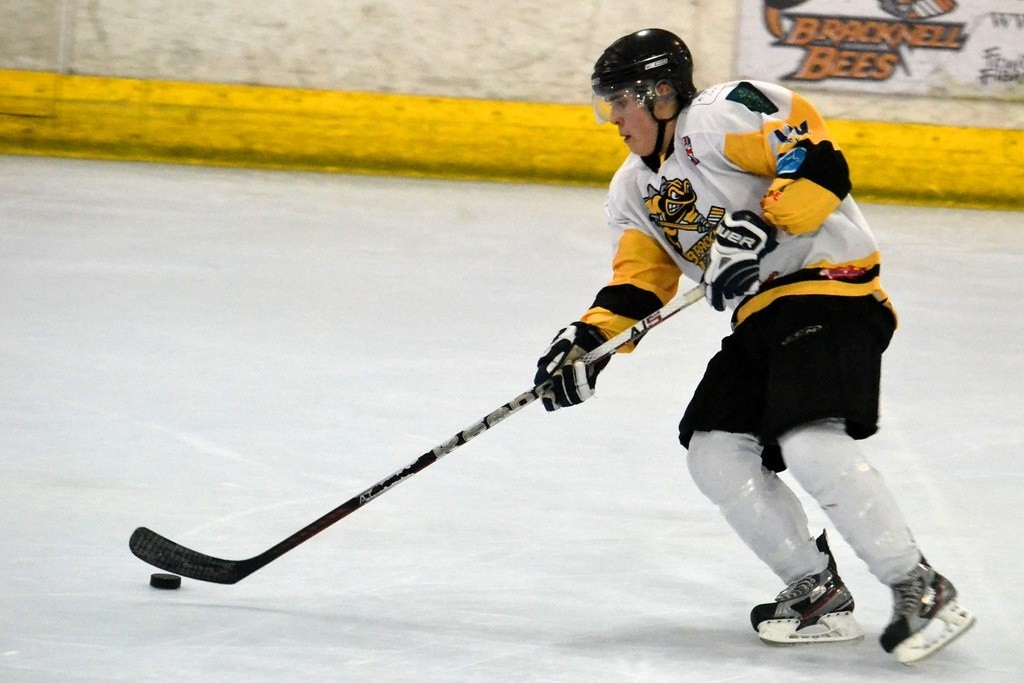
left=126, top=280, right=708, bottom=587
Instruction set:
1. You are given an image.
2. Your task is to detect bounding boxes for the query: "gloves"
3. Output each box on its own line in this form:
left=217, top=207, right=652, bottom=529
left=533, top=321, right=617, bottom=412
left=703, top=209, right=781, bottom=312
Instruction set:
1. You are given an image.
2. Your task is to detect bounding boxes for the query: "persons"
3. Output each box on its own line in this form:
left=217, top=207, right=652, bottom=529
left=534, top=26, right=974, bottom=668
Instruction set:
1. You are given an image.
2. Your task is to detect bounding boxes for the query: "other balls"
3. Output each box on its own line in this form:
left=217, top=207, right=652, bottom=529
left=148, top=572, right=184, bottom=591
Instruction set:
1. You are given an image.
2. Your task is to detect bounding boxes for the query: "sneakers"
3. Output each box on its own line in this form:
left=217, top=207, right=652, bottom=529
left=750, top=529, right=865, bottom=646
left=879, top=555, right=976, bottom=667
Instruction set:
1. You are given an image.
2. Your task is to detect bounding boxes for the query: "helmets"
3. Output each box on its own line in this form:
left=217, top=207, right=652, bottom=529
left=591, top=28, right=696, bottom=98
